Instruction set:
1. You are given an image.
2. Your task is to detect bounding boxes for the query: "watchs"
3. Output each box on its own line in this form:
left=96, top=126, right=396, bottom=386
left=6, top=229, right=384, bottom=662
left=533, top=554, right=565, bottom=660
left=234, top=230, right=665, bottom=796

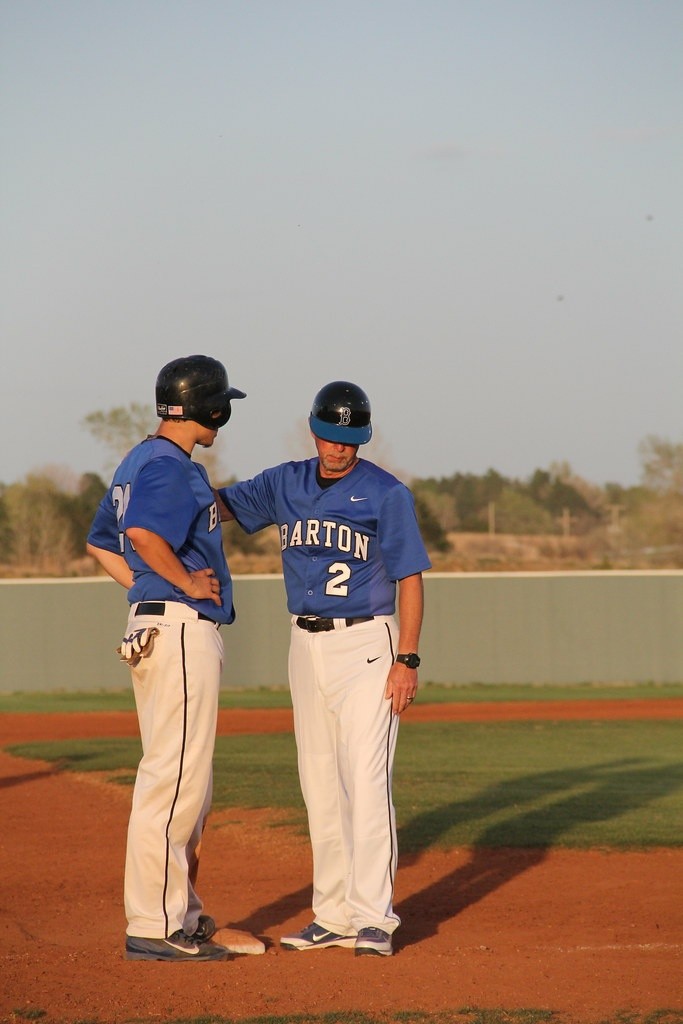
left=396, top=653, right=420, bottom=669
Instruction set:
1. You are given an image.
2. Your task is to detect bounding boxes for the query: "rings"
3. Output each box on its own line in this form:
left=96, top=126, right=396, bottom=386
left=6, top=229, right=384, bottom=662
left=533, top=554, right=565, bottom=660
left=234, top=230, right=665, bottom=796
left=407, top=697, right=413, bottom=700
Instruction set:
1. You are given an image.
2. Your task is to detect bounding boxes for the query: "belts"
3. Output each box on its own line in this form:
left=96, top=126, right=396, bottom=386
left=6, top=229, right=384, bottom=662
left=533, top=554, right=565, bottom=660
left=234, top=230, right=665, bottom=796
left=130, top=603, right=217, bottom=624
left=296, top=615, right=374, bottom=633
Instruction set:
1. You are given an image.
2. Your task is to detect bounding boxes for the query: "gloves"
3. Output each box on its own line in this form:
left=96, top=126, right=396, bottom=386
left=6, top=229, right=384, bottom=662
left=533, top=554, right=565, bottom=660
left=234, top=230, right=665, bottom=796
left=115, top=627, right=159, bottom=664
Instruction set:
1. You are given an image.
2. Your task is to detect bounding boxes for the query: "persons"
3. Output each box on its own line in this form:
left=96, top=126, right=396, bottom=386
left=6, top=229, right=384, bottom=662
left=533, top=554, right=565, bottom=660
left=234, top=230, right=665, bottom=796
left=87, top=355, right=247, bottom=962
left=209, top=381, right=432, bottom=958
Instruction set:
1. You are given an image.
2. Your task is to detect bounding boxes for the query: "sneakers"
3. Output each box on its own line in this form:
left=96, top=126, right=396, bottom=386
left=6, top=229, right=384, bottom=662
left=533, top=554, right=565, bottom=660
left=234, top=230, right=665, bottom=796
left=126, top=929, right=230, bottom=962
left=192, top=914, right=215, bottom=940
left=353, top=926, right=394, bottom=957
left=279, top=921, right=357, bottom=951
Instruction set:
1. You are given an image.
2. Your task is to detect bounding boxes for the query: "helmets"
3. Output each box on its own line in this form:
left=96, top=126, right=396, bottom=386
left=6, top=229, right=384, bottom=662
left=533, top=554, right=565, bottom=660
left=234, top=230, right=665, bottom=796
left=156, top=355, right=247, bottom=430
left=309, top=380, right=372, bottom=445
